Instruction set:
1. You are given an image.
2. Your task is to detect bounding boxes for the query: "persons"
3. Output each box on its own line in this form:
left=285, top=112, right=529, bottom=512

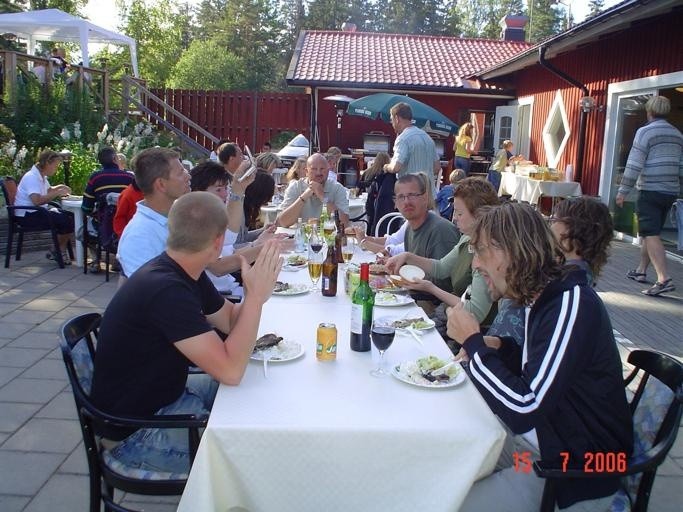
left=386, top=178, right=520, bottom=346
left=375, top=174, right=462, bottom=311
left=251, top=153, right=280, bottom=203
left=381, top=103, right=441, bottom=237
left=452, top=121, right=476, bottom=183
left=354, top=172, right=441, bottom=259
left=442, top=202, right=643, bottom=512
left=112, top=155, right=160, bottom=239
left=261, top=143, right=280, bottom=165
left=321, top=153, right=338, bottom=182
left=276, top=153, right=349, bottom=236
left=287, top=158, right=310, bottom=185
left=237, top=170, right=278, bottom=236
left=329, top=146, right=342, bottom=173
left=430, top=168, right=468, bottom=223
left=94, top=192, right=282, bottom=481
left=617, top=96, right=681, bottom=296
left=84, top=147, right=141, bottom=273
left=206, top=141, right=254, bottom=208
left=116, top=153, right=134, bottom=175
left=450, top=197, right=614, bottom=366
left=487, top=140, right=514, bottom=195
left=190, top=163, right=298, bottom=268
left=12, top=152, right=77, bottom=267
left=116, top=147, right=294, bottom=322
left=359, top=154, right=397, bottom=195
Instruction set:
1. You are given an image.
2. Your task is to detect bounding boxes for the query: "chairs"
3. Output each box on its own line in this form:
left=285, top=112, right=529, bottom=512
left=82, top=193, right=121, bottom=281
left=533, top=349, right=683, bottom=511
left=440, top=197, right=454, bottom=222
left=0, top=176, right=76, bottom=269
left=58, top=311, right=209, bottom=511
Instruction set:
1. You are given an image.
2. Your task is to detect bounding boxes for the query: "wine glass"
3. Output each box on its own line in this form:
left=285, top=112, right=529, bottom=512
left=311, top=237, right=321, bottom=259
left=323, top=220, right=335, bottom=239
left=308, top=253, right=321, bottom=290
left=372, top=318, right=394, bottom=375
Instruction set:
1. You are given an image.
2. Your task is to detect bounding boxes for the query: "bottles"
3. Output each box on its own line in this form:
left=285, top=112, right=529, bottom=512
left=321, top=244, right=337, bottom=297
left=318, top=201, right=331, bottom=237
left=350, top=264, right=372, bottom=352
left=316, top=321, right=338, bottom=362
left=294, top=217, right=305, bottom=255
left=335, top=224, right=348, bottom=263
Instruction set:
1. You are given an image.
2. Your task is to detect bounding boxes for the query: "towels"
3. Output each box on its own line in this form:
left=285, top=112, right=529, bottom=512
left=350, top=246, right=384, bottom=266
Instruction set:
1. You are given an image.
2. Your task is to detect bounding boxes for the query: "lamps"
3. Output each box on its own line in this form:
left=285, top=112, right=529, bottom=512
left=580, top=97, right=604, bottom=112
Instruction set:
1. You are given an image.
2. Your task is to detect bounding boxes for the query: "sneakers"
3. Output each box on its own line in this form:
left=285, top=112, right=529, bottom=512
left=641, top=277, right=677, bottom=295
left=626, top=269, right=646, bottom=283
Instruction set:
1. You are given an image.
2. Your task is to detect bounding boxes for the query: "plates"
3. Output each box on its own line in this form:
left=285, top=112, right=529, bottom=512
left=391, top=357, right=465, bottom=387
left=279, top=254, right=308, bottom=270
left=270, top=280, right=309, bottom=297
left=250, top=337, right=304, bottom=362
left=399, top=264, right=425, bottom=284
left=392, top=315, right=435, bottom=332
left=371, top=291, right=414, bottom=305
left=369, top=275, right=406, bottom=293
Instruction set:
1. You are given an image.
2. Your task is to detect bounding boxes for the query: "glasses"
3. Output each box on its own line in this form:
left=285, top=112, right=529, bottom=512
left=49, top=154, right=59, bottom=159
left=397, top=189, right=426, bottom=200
left=452, top=207, right=467, bottom=219
left=116, top=156, right=121, bottom=160
left=548, top=213, right=562, bottom=226
left=465, top=243, right=499, bottom=254
left=204, top=186, right=229, bottom=193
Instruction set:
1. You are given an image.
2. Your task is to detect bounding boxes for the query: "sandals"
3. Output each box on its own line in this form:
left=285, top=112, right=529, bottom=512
left=45, top=249, right=57, bottom=260
left=61, top=249, right=72, bottom=266
left=87, top=261, right=101, bottom=272
left=110, top=260, right=120, bottom=271
